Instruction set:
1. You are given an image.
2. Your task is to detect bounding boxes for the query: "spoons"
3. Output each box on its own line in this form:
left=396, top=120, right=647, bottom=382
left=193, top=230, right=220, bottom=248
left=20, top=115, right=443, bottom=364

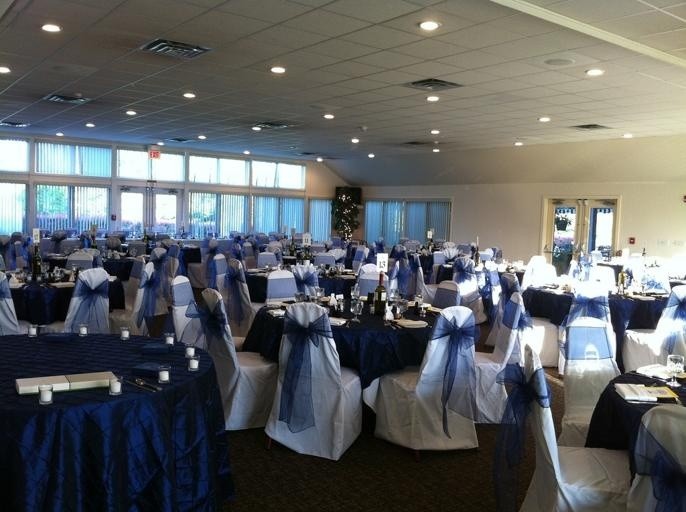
left=135, top=378, right=162, bottom=393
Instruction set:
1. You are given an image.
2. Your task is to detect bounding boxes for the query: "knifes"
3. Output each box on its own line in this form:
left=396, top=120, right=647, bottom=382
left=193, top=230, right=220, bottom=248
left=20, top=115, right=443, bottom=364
left=126, top=380, right=156, bottom=392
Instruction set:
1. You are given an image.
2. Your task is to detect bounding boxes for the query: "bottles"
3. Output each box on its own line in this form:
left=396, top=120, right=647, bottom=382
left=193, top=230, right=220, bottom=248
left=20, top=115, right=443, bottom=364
left=375, top=271, right=388, bottom=315
left=31, top=245, right=40, bottom=279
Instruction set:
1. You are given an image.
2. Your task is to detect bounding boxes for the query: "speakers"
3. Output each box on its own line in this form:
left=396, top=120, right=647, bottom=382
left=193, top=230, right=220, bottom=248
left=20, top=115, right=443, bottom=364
left=335, top=186, right=362, bottom=205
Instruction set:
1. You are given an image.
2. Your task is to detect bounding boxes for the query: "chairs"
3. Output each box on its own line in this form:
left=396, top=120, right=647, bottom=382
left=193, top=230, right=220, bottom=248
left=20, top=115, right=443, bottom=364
left=0, top=230, right=685, bottom=512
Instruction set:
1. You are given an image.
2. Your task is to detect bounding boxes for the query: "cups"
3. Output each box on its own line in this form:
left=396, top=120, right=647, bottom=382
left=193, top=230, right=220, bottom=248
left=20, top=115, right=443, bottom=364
left=158, top=366, right=171, bottom=383
left=38, top=383, right=53, bottom=404
left=108, top=376, right=124, bottom=396
left=164, top=333, right=174, bottom=344
left=7, top=268, right=77, bottom=284
left=188, top=356, right=199, bottom=371
left=120, top=326, right=130, bottom=341
left=291, top=287, right=428, bottom=320
left=28, top=324, right=38, bottom=337
left=185, top=344, right=195, bottom=358
left=78, top=324, right=88, bottom=336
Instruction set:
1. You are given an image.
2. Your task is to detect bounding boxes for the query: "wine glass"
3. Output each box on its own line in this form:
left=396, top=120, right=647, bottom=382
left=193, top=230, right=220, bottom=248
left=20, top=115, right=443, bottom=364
left=665, top=354, right=684, bottom=387
